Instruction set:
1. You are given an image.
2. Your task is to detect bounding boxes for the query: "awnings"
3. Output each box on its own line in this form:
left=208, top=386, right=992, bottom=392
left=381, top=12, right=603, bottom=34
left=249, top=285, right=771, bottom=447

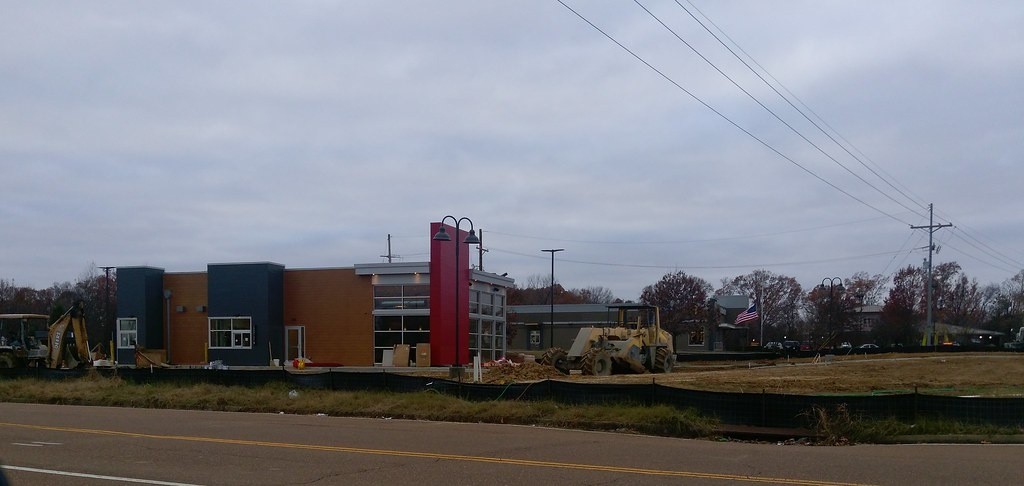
left=718, top=323, right=750, bottom=331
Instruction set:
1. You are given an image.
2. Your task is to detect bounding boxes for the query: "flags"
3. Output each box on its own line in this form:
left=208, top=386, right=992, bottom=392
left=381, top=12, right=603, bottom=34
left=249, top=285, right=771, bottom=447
left=736, top=303, right=758, bottom=324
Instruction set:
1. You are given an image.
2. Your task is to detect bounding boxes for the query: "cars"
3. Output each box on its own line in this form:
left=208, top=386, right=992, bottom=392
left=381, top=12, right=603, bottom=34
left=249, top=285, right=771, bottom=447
left=765, top=339, right=880, bottom=353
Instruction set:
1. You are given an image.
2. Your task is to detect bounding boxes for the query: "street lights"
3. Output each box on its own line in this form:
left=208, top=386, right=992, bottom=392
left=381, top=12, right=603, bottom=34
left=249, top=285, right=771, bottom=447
left=96, top=265, right=117, bottom=352
left=432, top=214, right=482, bottom=367
left=819, top=275, right=844, bottom=352
left=540, top=247, right=567, bottom=350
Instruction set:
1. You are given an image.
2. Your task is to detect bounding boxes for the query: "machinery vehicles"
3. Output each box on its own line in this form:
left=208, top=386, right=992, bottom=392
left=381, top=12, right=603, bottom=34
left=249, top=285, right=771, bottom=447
left=0, top=299, right=96, bottom=370
left=544, top=300, right=678, bottom=376
